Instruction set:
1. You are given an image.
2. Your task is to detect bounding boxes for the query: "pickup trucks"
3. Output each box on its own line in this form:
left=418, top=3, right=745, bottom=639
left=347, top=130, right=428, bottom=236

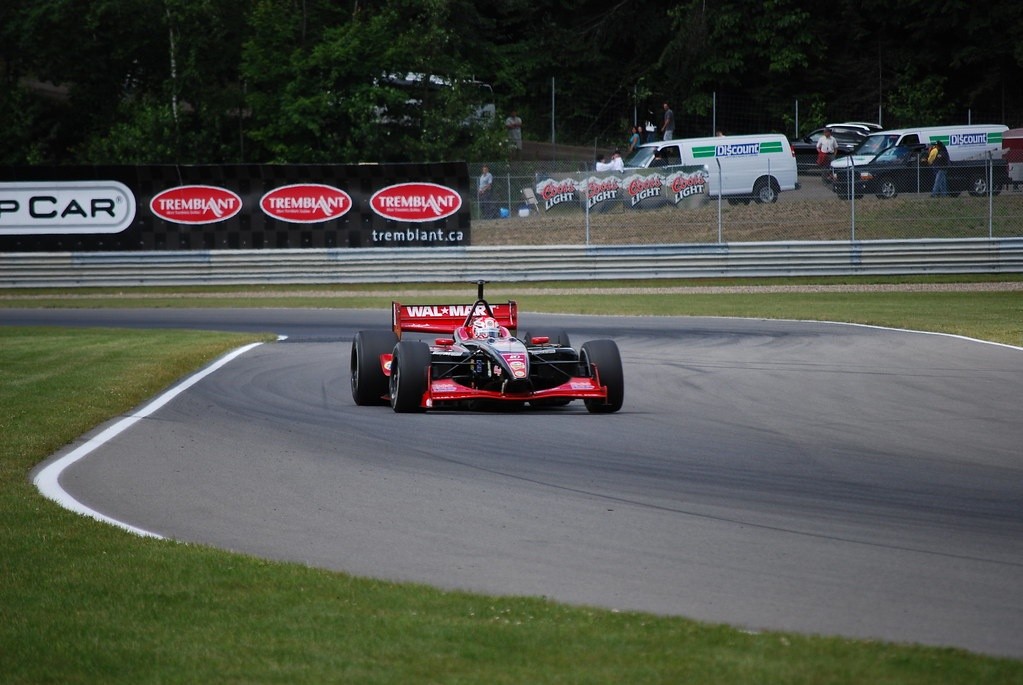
left=834, top=142, right=1009, bottom=200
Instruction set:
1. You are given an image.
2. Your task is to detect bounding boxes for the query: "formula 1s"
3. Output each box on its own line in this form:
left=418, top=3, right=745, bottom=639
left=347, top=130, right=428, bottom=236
left=350, top=276, right=625, bottom=413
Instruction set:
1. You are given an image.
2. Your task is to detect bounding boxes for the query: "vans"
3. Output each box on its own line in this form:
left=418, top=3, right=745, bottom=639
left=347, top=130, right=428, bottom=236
left=616, top=133, right=798, bottom=211
left=830, top=124, right=1009, bottom=199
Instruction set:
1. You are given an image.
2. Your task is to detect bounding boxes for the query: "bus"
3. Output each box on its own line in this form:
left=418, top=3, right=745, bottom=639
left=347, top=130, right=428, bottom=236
left=373, top=70, right=496, bottom=133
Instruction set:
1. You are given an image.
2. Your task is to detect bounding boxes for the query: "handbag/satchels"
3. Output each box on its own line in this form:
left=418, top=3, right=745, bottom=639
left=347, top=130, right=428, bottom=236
left=646, top=121, right=655, bottom=132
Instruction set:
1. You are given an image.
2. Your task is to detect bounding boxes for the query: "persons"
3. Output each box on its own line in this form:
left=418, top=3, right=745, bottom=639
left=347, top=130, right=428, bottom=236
left=919, top=140, right=951, bottom=197
left=505, top=109, right=523, bottom=152
left=661, top=103, right=674, bottom=154
left=628, top=126, right=645, bottom=154
left=595, top=150, right=624, bottom=171
left=478, top=164, right=493, bottom=220
left=471, top=316, right=501, bottom=339
left=644, top=104, right=657, bottom=143
left=816, top=128, right=838, bottom=186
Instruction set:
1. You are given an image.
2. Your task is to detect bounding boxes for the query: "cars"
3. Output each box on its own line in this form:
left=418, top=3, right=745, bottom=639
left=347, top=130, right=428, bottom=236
left=822, top=121, right=886, bottom=156
left=786, top=127, right=867, bottom=175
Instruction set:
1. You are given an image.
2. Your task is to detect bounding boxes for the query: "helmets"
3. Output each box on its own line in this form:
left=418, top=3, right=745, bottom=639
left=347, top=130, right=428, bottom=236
left=472, top=316, right=501, bottom=340
